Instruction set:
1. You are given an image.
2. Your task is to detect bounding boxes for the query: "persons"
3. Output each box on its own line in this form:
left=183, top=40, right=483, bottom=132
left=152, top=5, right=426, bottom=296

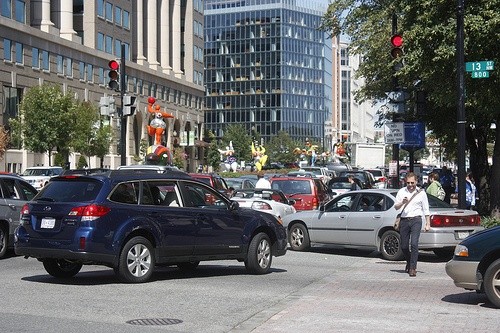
left=320, top=152, right=330, bottom=166
left=254, top=171, right=273, bottom=194
left=400, top=165, right=409, bottom=174
left=217, top=146, right=238, bottom=172
left=149, top=186, right=160, bottom=205
left=252, top=142, right=268, bottom=171
left=147, top=103, right=174, bottom=144
left=83, top=163, right=88, bottom=169
left=196, top=162, right=213, bottom=173
left=348, top=176, right=360, bottom=203
left=63, top=163, right=69, bottom=170
left=295, top=137, right=313, bottom=167
left=394, top=172, right=430, bottom=277
left=425, top=166, right=479, bottom=210
left=333, top=139, right=351, bottom=163
left=223, top=187, right=237, bottom=198
left=419, top=169, right=428, bottom=185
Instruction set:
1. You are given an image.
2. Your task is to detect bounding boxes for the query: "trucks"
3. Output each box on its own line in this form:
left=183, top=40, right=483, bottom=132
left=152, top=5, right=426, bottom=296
left=351, top=144, right=386, bottom=169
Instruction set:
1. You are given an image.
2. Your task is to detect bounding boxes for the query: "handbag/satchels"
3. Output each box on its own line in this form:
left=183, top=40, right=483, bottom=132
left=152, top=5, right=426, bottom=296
left=393, top=214, right=401, bottom=231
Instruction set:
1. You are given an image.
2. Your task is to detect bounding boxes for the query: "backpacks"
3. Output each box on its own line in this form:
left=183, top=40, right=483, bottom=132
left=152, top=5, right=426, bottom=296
left=437, top=186, right=446, bottom=201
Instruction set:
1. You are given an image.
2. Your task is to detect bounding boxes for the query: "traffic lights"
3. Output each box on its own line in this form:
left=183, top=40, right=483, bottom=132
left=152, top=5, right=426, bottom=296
left=108, top=59, right=121, bottom=92
left=390, top=34, right=404, bottom=71
left=122, top=96, right=136, bottom=117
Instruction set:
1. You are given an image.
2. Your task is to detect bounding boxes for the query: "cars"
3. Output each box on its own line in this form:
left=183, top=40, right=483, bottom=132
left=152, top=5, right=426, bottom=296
left=279, top=188, right=485, bottom=262
left=226, top=187, right=297, bottom=218
left=444, top=225, right=500, bottom=309
left=0, top=172, right=38, bottom=260
left=114, top=163, right=443, bottom=219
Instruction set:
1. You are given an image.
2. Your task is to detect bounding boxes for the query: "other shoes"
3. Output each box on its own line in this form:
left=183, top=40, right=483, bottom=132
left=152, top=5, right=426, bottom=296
left=409, top=268, right=416, bottom=276
left=405, top=265, right=410, bottom=272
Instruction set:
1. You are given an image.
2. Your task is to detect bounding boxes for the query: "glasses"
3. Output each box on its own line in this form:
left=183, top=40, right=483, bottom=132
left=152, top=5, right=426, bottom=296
left=406, top=182, right=415, bottom=185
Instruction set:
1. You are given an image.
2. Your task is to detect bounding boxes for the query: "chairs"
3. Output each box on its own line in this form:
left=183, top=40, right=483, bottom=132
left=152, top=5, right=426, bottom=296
left=164, top=192, right=177, bottom=207
left=235, top=193, right=272, bottom=200
left=360, top=197, right=370, bottom=210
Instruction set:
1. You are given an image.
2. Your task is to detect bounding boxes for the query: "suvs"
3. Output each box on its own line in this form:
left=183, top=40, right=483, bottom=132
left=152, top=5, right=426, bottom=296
left=19, top=167, right=62, bottom=192
left=13, top=167, right=288, bottom=284
